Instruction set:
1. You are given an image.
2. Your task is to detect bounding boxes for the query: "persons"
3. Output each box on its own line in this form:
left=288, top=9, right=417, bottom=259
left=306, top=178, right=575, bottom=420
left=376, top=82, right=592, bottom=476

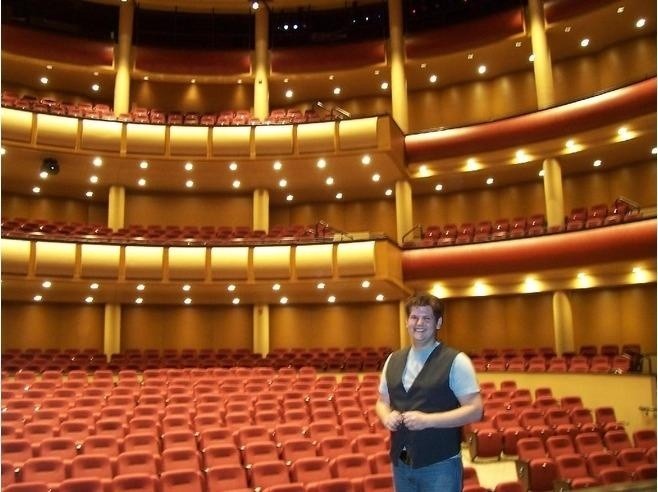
left=375, top=294, right=484, bottom=492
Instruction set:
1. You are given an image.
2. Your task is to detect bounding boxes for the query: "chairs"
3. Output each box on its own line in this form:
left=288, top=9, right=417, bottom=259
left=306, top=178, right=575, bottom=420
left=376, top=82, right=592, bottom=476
left=1, top=215, right=335, bottom=246
left=1, top=344, right=656, bottom=492
left=423, top=199, right=645, bottom=248
left=1, top=87, right=339, bottom=127
